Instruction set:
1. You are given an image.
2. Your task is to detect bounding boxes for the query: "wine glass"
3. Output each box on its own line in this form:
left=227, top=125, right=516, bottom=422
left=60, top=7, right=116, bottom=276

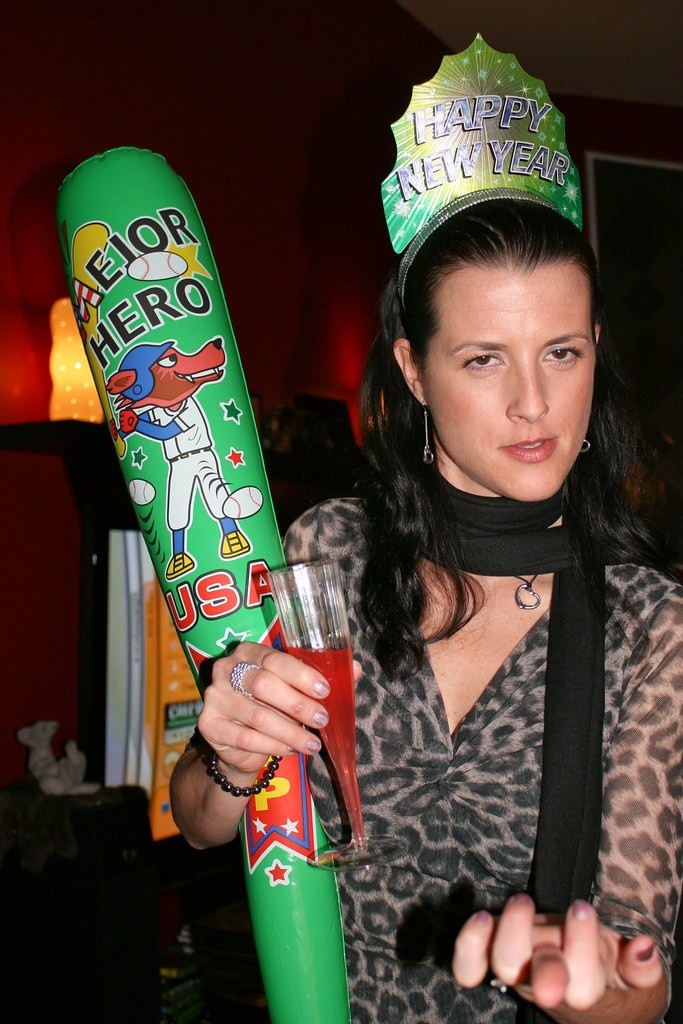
left=267, top=559, right=408, bottom=872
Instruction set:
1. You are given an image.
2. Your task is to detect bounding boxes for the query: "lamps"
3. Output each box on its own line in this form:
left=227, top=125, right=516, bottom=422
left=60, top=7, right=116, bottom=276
left=46, top=297, right=106, bottom=421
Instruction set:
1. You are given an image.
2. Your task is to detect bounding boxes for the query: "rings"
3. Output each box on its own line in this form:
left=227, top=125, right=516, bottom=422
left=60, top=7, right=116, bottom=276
left=491, top=978, right=506, bottom=992
left=230, top=661, right=260, bottom=698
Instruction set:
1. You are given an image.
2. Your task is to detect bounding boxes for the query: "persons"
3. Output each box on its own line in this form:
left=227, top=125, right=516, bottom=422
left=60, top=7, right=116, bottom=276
left=171, top=199, right=683, bottom=1024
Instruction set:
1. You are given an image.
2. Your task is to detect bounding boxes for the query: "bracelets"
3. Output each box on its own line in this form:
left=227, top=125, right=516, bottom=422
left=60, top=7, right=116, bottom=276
left=207, top=750, right=283, bottom=798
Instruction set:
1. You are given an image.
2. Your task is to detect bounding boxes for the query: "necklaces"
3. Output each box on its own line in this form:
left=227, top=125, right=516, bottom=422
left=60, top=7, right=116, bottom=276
left=514, top=575, right=540, bottom=609
